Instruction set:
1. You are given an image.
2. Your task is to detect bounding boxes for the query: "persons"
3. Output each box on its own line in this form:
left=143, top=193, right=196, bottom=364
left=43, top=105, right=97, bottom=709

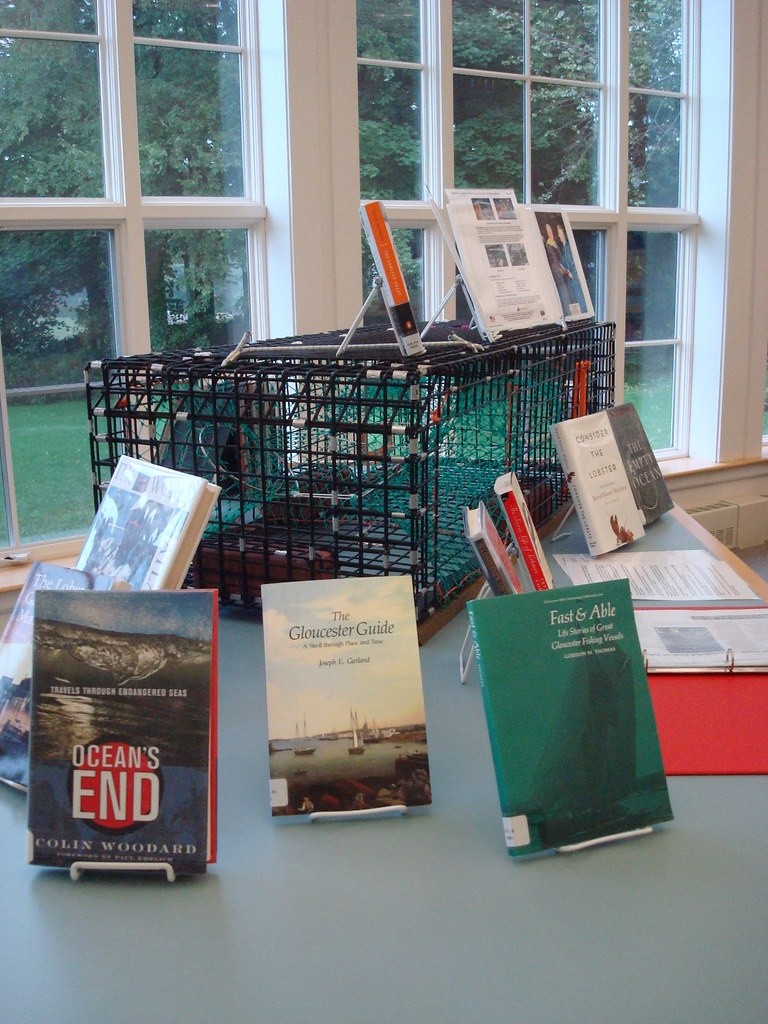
left=546, top=221, right=588, bottom=316
left=295, top=767, right=429, bottom=814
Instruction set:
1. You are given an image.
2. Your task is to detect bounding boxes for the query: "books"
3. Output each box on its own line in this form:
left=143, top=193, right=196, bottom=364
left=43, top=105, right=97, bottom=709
left=363, top=199, right=425, bottom=357
left=0, top=561, right=132, bottom=795
left=463, top=501, right=526, bottom=596
left=464, top=576, right=675, bottom=857
left=548, top=400, right=675, bottom=558
left=79, top=455, right=222, bottom=590
left=25, top=587, right=218, bottom=875
left=260, top=574, right=434, bottom=822
left=437, top=181, right=600, bottom=359
left=493, top=470, right=555, bottom=592
